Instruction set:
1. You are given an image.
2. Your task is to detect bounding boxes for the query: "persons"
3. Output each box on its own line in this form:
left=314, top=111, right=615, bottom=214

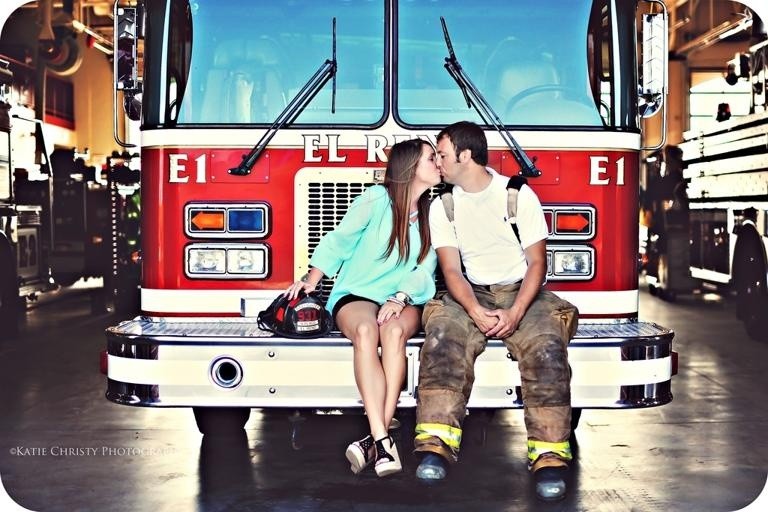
left=412, top=121, right=579, bottom=501
left=283, top=139, right=441, bottom=478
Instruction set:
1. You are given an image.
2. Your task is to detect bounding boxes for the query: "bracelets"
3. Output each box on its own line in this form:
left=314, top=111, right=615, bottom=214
left=386, top=297, right=406, bottom=307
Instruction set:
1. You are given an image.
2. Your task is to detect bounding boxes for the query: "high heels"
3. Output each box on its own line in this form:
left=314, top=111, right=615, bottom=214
left=345, top=435, right=402, bottom=478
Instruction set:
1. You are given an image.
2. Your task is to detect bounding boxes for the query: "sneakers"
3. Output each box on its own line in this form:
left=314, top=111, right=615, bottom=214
left=415, top=447, right=449, bottom=482
left=536, top=464, right=570, bottom=502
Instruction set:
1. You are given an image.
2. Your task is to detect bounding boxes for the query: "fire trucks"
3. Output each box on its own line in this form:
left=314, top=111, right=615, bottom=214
left=100, top=0, right=678, bottom=436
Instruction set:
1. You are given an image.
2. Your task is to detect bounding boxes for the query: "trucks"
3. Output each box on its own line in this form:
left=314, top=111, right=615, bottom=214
left=648, top=107, right=767, bottom=342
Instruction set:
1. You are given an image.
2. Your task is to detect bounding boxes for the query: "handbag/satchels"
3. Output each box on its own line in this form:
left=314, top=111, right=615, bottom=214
left=257, top=287, right=332, bottom=339
